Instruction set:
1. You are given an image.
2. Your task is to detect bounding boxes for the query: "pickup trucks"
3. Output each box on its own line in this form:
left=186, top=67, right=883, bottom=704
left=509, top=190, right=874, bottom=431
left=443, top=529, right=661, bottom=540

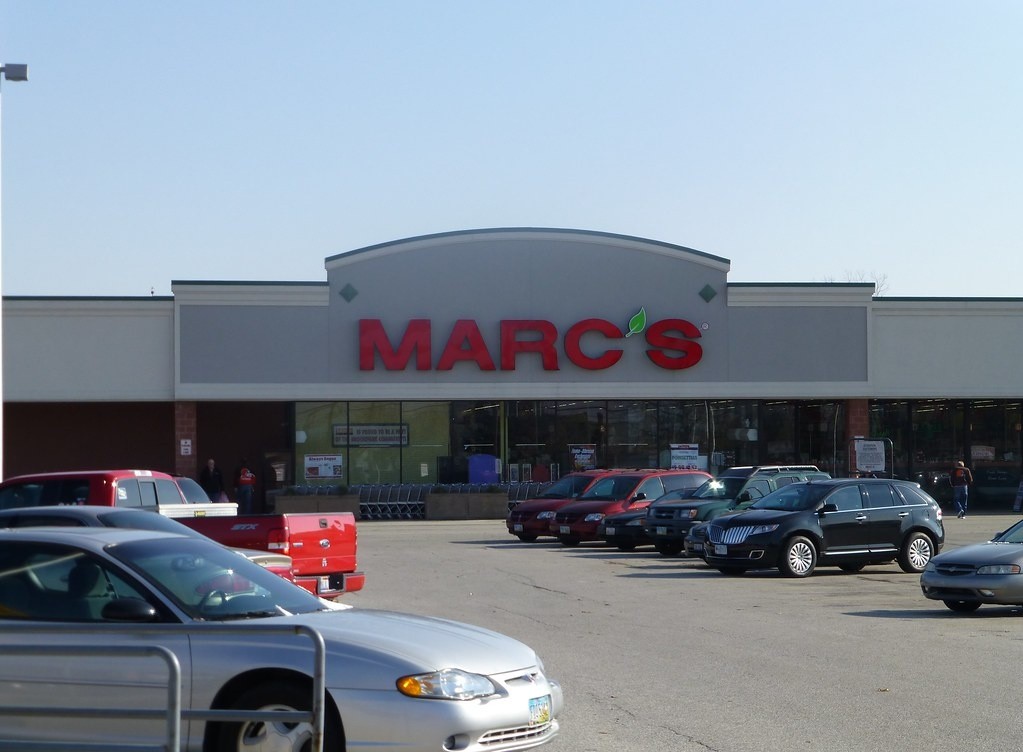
left=0, top=469, right=365, bottom=603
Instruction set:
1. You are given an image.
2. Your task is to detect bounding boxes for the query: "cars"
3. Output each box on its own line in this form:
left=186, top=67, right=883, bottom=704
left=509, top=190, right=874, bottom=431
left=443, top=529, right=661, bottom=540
left=683, top=519, right=711, bottom=566
left=548, top=468, right=713, bottom=547
left=0, top=526, right=563, bottom=751
left=506, top=468, right=635, bottom=543
left=597, top=485, right=696, bottom=556
left=0, top=503, right=292, bottom=609
left=918, top=518, right=1023, bottom=613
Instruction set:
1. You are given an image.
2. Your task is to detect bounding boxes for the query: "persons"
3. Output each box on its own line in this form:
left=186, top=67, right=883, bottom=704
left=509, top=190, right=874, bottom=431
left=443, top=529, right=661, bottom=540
left=243, top=452, right=271, bottom=511
left=201, top=460, right=222, bottom=503
left=950, top=459, right=972, bottom=520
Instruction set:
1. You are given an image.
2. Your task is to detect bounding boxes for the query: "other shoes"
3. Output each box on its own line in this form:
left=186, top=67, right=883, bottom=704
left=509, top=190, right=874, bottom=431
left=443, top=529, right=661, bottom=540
left=958, top=509, right=964, bottom=518
left=962, top=515, right=966, bottom=519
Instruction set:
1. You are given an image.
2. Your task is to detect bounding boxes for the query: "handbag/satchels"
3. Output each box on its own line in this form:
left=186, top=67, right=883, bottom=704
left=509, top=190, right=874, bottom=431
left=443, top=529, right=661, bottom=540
left=218, top=491, right=230, bottom=503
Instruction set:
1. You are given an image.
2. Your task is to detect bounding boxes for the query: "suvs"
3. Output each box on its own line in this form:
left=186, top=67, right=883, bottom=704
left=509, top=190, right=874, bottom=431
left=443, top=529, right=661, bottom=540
left=684, top=474, right=945, bottom=578
left=643, top=464, right=832, bottom=556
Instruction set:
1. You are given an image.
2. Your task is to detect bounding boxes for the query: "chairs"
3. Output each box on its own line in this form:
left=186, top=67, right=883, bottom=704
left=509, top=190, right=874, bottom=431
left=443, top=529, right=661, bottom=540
left=59, top=566, right=119, bottom=619
